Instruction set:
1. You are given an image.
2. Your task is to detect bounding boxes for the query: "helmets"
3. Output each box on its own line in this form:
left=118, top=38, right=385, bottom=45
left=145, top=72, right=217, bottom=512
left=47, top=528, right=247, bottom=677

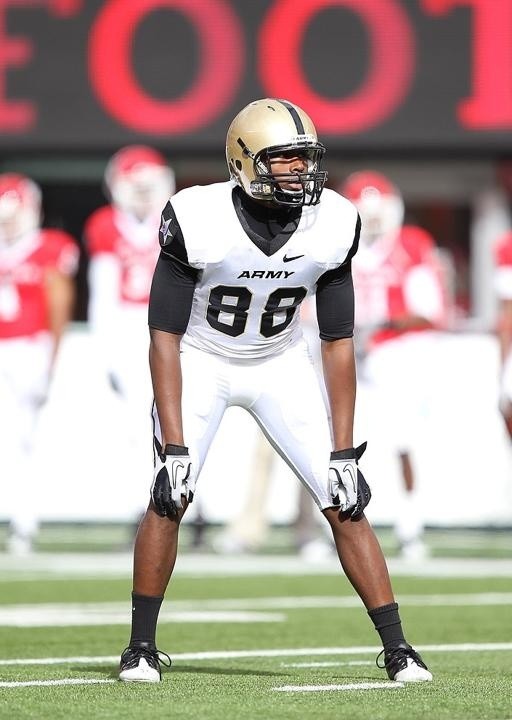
left=335, top=170, right=406, bottom=262
left=104, top=145, right=176, bottom=225
left=0, top=174, right=44, bottom=245
left=224, top=98, right=329, bottom=210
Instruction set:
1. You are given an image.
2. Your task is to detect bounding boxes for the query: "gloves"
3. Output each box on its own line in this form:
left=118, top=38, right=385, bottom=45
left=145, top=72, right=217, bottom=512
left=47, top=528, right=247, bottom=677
left=150, top=444, right=197, bottom=521
left=328, top=441, right=372, bottom=521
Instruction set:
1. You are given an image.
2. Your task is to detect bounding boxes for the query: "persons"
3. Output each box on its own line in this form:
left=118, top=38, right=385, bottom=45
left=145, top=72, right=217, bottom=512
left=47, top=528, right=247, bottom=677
left=115, top=92, right=438, bottom=687
left=493, top=230, right=512, bottom=443
left=2, top=169, right=80, bottom=557
left=84, top=142, right=180, bottom=401
left=299, top=168, right=462, bottom=568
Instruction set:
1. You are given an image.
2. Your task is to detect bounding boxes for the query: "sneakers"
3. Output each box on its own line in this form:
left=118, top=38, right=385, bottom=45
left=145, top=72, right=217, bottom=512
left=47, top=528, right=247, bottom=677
left=119, top=640, right=172, bottom=683
left=376, top=641, right=433, bottom=682
